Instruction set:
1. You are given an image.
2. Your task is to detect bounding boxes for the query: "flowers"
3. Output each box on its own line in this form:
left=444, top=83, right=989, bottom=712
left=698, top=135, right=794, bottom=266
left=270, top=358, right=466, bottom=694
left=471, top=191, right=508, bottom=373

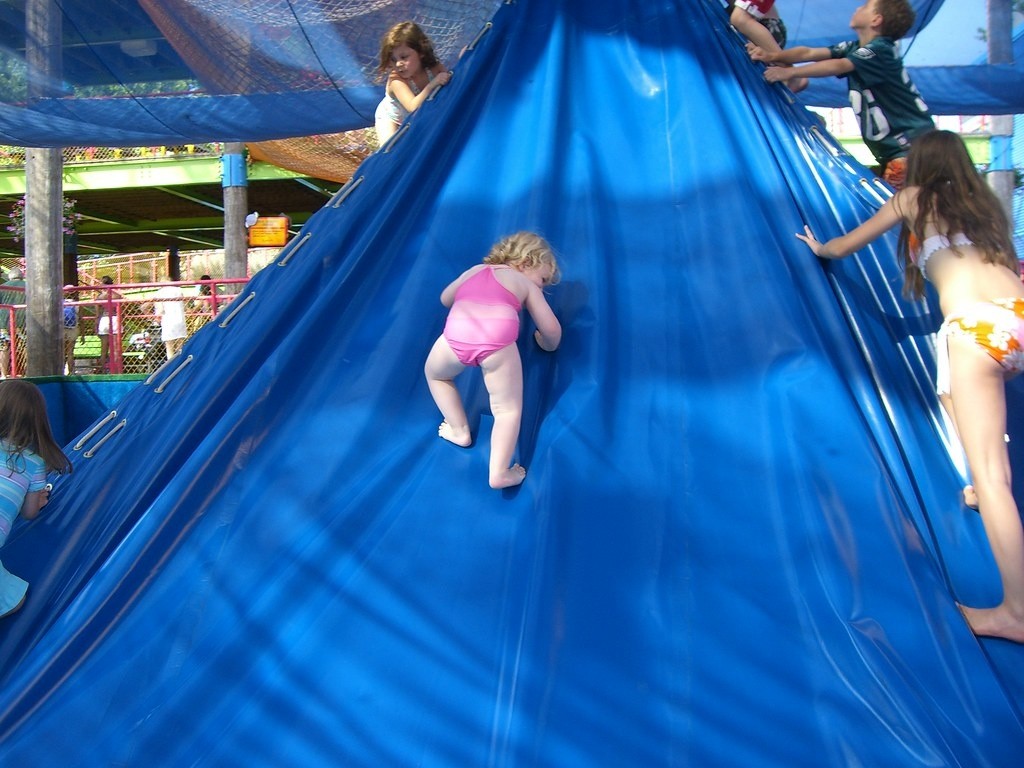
left=6, top=191, right=78, bottom=244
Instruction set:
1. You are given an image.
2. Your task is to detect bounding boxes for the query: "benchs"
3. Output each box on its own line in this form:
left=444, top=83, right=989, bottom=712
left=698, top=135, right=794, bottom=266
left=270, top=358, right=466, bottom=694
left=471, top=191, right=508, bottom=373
left=66, top=333, right=158, bottom=372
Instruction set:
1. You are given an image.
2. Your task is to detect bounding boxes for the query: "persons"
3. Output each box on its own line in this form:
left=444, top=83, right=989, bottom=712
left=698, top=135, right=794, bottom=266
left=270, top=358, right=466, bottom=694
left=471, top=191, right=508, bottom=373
left=423, top=231, right=561, bottom=488
left=0, top=379, right=72, bottom=617
left=724, top=0, right=936, bottom=191
left=375, top=20, right=451, bottom=149
left=0, top=327, right=10, bottom=380
left=192, top=275, right=224, bottom=330
left=794, top=128, right=1024, bottom=643
left=61, top=284, right=85, bottom=377
left=93, top=275, right=123, bottom=374
left=141, top=276, right=186, bottom=361
